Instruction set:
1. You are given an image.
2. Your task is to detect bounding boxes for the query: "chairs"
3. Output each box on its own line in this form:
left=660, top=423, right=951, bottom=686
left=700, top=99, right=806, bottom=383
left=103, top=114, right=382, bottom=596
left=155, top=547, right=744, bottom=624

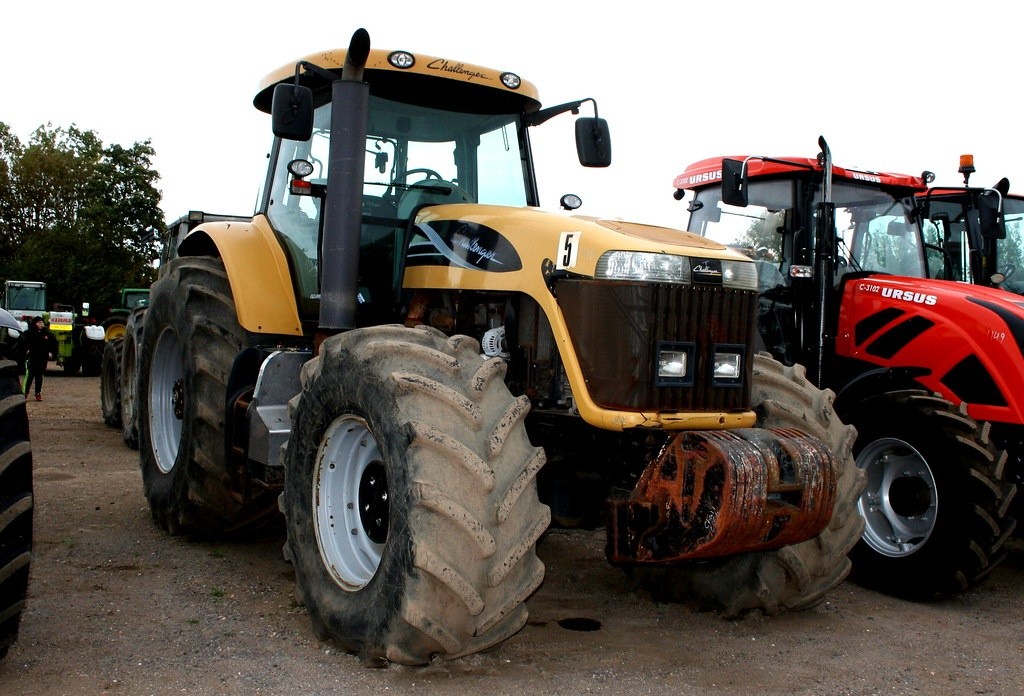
left=360, top=196, right=397, bottom=246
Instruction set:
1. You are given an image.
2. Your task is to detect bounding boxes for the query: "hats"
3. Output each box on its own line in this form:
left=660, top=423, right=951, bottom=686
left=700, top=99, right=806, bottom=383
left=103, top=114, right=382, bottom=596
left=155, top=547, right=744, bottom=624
left=32, top=316, right=44, bottom=324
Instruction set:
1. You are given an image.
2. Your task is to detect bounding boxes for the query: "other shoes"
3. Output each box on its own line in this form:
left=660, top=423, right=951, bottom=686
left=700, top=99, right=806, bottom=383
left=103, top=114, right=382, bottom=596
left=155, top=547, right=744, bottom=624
left=36, top=393, right=43, bottom=401
left=25, top=395, right=28, bottom=402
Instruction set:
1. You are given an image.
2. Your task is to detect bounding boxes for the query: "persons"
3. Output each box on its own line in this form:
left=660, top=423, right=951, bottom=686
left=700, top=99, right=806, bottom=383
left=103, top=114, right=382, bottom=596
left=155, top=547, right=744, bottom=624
left=14, top=316, right=57, bottom=401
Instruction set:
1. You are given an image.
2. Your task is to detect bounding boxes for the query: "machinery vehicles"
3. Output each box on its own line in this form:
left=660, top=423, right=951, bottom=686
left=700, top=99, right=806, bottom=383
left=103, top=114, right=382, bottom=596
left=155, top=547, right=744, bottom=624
left=133, top=28, right=866, bottom=666
left=671, top=135, right=1024, bottom=607
left=0, top=307, right=35, bottom=663
left=1, top=210, right=257, bottom=430
